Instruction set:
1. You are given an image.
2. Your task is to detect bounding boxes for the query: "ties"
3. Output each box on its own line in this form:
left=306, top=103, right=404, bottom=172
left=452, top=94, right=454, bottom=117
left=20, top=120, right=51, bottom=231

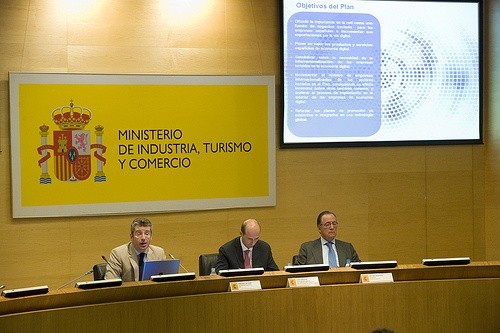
left=326, top=243, right=337, bottom=267
left=138, top=253, right=145, bottom=282
left=244, top=250, right=251, bottom=268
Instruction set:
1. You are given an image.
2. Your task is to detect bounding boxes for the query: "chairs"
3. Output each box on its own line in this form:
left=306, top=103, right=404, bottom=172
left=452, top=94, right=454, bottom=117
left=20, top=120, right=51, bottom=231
left=198, top=254, right=218, bottom=276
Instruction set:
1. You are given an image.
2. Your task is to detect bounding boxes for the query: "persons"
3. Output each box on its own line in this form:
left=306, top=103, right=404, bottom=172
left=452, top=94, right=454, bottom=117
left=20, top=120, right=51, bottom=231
left=296, top=211, right=363, bottom=267
left=217, top=218, right=279, bottom=271
left=104, top=218, right=167, bottom=282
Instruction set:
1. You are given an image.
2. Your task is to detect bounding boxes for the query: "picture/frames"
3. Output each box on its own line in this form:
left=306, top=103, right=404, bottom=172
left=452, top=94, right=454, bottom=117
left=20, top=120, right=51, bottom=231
left=8, top=72, right=277, bottom=218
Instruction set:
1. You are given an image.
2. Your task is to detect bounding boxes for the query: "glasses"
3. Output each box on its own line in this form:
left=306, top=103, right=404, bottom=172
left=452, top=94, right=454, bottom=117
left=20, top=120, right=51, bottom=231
left=320, top=221, right=338, bottom=227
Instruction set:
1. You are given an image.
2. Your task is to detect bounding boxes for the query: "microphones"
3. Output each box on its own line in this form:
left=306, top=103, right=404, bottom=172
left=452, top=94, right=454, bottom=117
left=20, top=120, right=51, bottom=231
left=169, top=254, right=189, bottom=273
left=0, top=285, right=6, bottom=289
left=57, top=270, right=95, bottom=291
left=101, top=256, right=121, bottom=279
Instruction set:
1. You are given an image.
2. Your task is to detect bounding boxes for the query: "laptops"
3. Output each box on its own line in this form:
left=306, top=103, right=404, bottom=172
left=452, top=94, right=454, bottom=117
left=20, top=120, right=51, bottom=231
left=142, top=259, right=181, bottom=281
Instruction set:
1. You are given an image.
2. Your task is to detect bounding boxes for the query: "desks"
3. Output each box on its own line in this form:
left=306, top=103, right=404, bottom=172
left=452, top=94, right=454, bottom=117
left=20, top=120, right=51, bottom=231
left=0, top=261, right=500, bottom=333
